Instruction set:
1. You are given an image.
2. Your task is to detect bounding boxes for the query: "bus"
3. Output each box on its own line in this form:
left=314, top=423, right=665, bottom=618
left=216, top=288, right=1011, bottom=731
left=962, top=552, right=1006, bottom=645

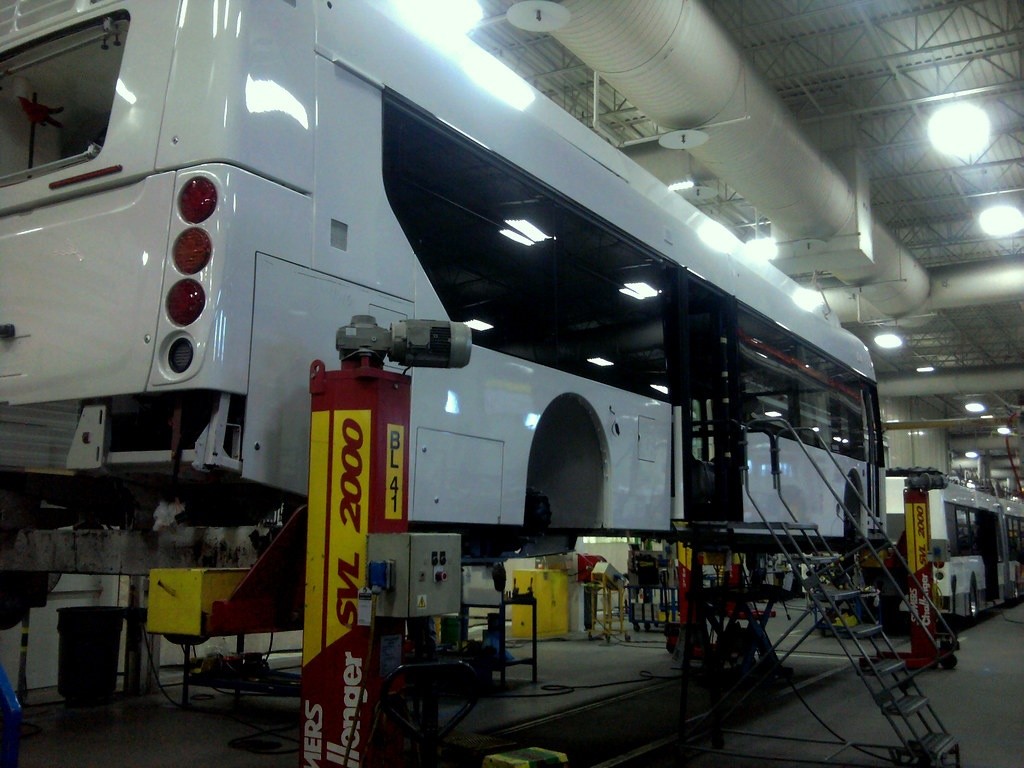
left=0, top=0, right=886, bottom=541
left=886, top=477, right=1024, bottom=607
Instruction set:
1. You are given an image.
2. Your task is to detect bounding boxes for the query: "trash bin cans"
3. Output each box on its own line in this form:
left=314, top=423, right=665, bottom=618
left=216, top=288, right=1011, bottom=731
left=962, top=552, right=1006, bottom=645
left=56, top=604, right=125, bottom=702
